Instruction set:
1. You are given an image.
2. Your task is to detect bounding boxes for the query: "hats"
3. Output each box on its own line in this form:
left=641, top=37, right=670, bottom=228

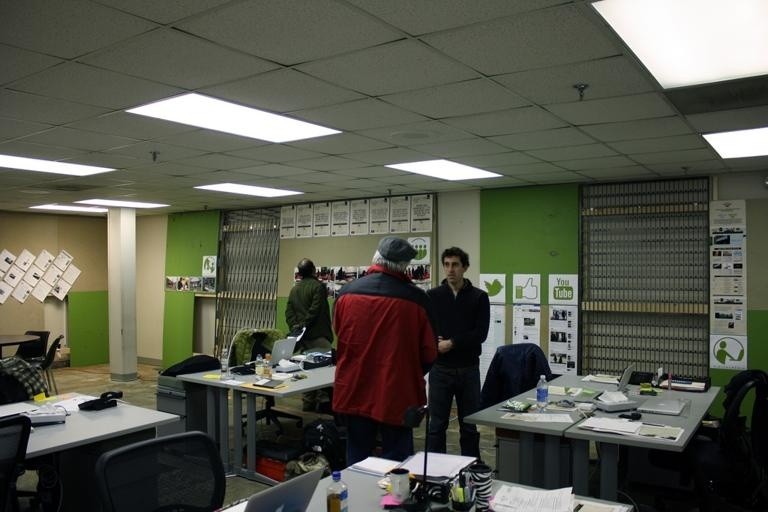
left=377, top=236, right=418, bottom=262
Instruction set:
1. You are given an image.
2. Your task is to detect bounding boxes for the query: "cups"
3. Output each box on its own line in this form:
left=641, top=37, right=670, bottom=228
left=451, top=464, right=492, bottom=512
left=389, top=467, right=411, bottom=502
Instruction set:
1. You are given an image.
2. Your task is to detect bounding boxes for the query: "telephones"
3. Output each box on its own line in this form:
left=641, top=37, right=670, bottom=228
left=78, top=391, right=122, bottom=410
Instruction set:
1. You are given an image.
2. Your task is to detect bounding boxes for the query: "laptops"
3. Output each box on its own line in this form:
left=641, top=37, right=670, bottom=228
left=637, top=398, right=685, bottom=415
left=262, top=338, right=296, bottom=366
left=220, top=466, right=324, bottom=512
left=617, top=364, right=634, bottom=391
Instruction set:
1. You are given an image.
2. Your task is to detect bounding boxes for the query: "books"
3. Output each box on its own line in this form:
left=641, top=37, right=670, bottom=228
left=346, top=456, right=401, bottom=478
left=252, top=378, right=286, bottom=390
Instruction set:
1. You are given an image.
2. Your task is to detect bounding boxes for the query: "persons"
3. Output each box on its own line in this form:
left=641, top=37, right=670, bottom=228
left=330, top=234, right=441, bottom=467
left=283, top=256, right=335, bottom=414
left=424, top=246, right=491, bottom=468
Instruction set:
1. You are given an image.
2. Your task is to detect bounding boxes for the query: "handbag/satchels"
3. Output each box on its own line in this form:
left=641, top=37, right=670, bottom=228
left=286, top=452, right=329, bottom=474
left=303, top=419, right=341, bottom=471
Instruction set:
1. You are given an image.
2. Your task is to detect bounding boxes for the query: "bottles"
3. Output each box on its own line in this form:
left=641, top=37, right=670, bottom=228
left=536, top=374, right=549, bottom=412
left=255, top=353, right=273, bottom=382
left=326, top=470, right=349, bottom=511
left=221, top=349, right=230, bottom=379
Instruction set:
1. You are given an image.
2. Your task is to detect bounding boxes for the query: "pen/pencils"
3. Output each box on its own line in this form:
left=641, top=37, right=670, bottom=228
left=642, top=422, right=665, bottom=428
